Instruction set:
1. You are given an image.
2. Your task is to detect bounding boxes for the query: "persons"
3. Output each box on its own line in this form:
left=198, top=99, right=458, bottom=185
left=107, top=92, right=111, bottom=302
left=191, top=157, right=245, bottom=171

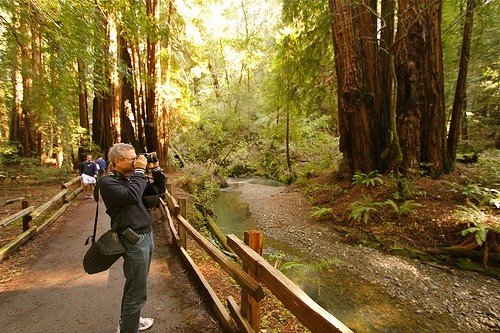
left=99, top=143, right=166, bottom=333
left=81, top=155, right=106, bottom=199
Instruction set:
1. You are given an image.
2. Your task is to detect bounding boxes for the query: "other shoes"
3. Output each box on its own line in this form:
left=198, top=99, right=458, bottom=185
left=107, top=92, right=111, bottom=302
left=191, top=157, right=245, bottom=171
left=84, top=194, right=93, bottom=200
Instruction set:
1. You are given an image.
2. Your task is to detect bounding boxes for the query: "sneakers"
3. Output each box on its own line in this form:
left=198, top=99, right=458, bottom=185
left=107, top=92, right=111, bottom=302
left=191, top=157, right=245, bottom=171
left=118, top=317, right=153, bottom=331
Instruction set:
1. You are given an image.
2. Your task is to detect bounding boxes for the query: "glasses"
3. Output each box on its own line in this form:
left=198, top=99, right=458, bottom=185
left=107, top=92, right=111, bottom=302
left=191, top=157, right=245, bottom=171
left=123, top=156, right=138, bottom=162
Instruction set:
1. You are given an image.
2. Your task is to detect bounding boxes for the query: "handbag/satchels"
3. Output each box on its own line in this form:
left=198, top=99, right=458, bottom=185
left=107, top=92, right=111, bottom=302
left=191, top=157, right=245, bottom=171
left=82, top=229, right=125, bottom=274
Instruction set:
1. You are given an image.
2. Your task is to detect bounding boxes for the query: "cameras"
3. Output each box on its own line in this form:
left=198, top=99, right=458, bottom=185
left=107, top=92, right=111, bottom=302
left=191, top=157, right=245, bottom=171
left=138, top=153, right=158, bottom=162
